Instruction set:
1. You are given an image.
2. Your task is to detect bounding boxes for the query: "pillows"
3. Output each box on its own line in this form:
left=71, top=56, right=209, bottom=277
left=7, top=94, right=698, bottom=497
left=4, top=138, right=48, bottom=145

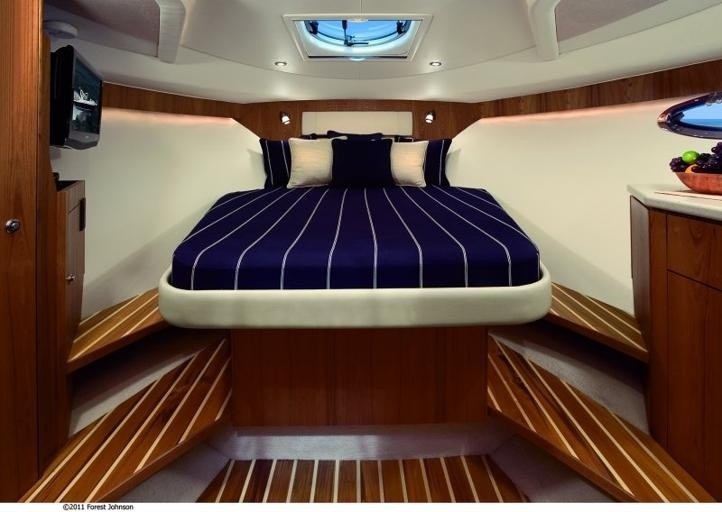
left=263, top=129, right=452, bottom=191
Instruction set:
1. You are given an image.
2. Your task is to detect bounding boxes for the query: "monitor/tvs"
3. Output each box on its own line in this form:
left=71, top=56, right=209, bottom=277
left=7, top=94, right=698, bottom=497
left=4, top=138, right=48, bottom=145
left=50, top=45, right=103, bottom=150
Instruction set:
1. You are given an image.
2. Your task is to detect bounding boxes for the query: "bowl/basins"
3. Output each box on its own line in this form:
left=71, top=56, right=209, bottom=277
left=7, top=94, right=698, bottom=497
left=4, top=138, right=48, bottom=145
left=673, top=171, right=721, bottom=196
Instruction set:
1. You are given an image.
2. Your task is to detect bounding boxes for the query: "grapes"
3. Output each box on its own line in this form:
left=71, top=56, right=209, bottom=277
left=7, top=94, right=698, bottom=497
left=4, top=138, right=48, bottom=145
left=669, top=157, right=686, bottom=172
left=691, top=142, right=722, bottom=171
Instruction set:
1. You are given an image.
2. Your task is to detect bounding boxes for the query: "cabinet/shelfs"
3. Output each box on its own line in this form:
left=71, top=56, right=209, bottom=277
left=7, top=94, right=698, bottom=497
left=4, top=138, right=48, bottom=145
left=45, top=170, right=86, bottom=379
left=626, top=185, right=720, bottom=496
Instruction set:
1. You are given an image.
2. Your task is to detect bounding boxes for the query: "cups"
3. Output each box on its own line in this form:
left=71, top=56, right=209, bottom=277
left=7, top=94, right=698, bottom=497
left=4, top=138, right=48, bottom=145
left=53, top=171, right=59, bottom=188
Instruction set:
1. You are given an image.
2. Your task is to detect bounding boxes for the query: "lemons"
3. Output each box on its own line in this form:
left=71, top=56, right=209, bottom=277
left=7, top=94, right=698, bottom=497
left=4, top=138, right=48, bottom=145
left=683, top=150, right=699, bottom=164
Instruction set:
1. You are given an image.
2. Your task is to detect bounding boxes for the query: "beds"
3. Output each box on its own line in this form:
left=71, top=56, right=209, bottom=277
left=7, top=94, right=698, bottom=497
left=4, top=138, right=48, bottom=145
left=159, top=182, right=549, bottom=461
